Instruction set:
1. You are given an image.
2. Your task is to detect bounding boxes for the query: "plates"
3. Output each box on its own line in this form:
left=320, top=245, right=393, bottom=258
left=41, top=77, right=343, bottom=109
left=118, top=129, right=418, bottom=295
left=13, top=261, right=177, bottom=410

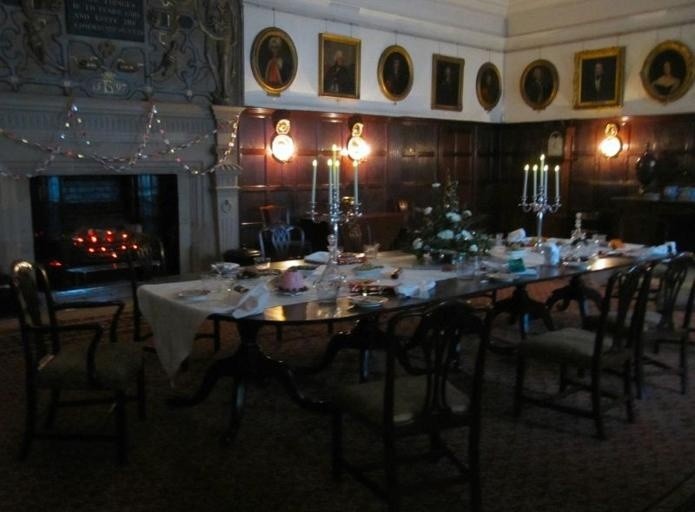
left=176, top=289, right=209, bottom=302
left=348, top=295, right=389, bottom=308
left=352, top=279, right=402, bottom=290
left=352, top=267, right=384, bottom=277
left=263, top=277, right=312, bottom=297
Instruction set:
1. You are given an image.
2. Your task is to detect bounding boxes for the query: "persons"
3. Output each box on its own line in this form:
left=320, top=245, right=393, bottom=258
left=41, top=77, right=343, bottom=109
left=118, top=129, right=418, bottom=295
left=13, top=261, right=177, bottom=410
left=583, top=63, right=614, bottom=100
left=384, top=58, right=408, bottom=96
left=324, top=50, right=354, bottom=94
left=259, top=36, right=291, bottom=86
left=527, top=67, right=552, bottom=103
left=435, top=65, right=458, bottom=106
left=650, top=59, right=681, bottom=96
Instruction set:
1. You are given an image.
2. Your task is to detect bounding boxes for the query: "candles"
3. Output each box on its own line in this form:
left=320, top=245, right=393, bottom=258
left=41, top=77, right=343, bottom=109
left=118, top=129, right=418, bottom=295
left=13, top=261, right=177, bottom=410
left=520, top=152, right=562, bottom=205
left=307, top=144, right=364, bottom=214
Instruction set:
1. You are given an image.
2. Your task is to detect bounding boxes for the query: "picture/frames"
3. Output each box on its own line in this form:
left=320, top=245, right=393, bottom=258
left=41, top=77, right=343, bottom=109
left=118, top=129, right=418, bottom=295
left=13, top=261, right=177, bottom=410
left=518, top=58, right=560, bottom=112
left=638, top=39, right=695, bottom=107
left=316, top=32, right=363, bottom=101
left=375, top=44, right=416, bottom=103
left=474, top=61, right=503, bottom=114
left=570, top=44, right=628, bottom=112
left=247, top=26, right=300, bottom=98
left=428, top=52, right=466, bottom=113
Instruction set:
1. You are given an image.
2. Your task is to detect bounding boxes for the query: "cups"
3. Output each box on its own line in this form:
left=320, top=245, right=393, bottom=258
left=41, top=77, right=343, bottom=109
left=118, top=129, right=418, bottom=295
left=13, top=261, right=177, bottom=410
left=253, top=257, right=272, bottom=272
left=199, top=270, right=235, bottom=303
left=361, top=243, right=382, bottom=264
left=664, top=185, right=678, bottom=200
left=316, top=281, right=339, bottom=305
left=455, top=262, right=475, bottom=281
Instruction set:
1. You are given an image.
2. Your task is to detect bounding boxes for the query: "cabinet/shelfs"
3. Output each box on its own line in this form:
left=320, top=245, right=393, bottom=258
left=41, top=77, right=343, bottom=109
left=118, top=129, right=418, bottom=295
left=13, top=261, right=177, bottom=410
left=610, top=191, right=695, bottom=271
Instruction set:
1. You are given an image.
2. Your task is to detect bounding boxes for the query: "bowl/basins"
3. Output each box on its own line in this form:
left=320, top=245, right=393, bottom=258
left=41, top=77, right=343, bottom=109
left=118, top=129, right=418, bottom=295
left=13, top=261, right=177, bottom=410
left=297, top=266, right=322, bottom=276
left=507, top=250, right=525, bottom=260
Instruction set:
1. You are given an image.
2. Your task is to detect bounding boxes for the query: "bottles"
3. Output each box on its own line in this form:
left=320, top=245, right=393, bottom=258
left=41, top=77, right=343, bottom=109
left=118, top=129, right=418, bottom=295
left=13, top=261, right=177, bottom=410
left=319, top=234, right=340, bottom=287
